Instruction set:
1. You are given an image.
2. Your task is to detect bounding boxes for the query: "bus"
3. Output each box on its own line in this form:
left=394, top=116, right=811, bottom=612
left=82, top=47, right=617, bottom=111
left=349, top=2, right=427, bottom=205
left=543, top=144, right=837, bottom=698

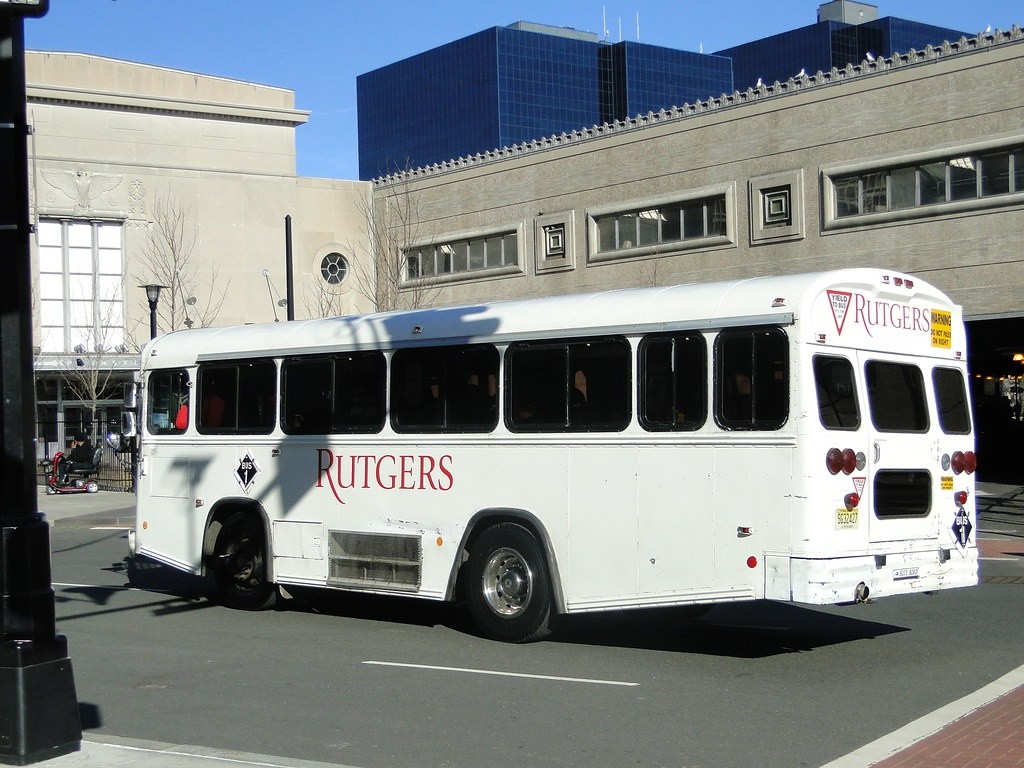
left=127, top=267, right=979, bottom=647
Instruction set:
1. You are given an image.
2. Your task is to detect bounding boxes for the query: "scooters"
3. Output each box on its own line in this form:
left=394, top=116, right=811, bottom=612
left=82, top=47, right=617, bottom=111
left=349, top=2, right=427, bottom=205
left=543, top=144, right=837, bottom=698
left=44, top=445, right=102, bottom=495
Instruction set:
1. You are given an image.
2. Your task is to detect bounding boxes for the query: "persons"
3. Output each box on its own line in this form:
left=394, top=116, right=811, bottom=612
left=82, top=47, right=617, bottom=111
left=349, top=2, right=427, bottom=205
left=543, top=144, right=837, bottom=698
left=175, top=386, right=224, bottom=429
left=59, top=434, right=95, bottom=482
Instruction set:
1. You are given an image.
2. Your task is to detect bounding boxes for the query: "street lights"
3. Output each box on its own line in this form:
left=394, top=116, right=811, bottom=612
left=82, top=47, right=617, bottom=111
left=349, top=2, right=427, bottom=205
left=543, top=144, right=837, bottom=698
left=136, top=283, right=171, bottom=341
